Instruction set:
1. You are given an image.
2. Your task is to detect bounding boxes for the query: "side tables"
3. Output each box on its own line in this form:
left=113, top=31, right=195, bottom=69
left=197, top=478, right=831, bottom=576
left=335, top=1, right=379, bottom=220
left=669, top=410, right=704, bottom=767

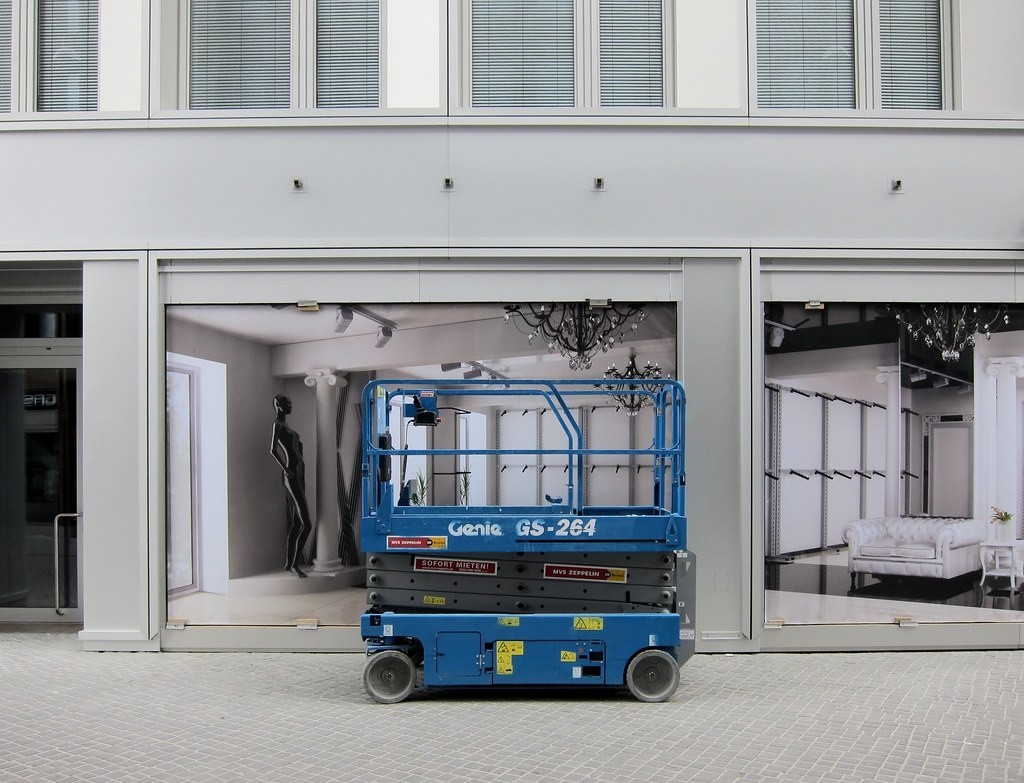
left=978, top=540, right=1024, bottom=592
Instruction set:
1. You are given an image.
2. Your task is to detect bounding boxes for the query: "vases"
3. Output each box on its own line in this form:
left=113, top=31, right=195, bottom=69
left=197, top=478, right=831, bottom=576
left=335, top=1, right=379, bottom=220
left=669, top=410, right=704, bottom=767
left=995, top=524, right=1017, bottom=541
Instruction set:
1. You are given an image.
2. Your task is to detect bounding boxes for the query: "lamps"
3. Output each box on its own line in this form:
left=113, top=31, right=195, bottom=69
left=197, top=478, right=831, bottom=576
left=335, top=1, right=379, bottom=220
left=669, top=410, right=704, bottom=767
left=333, top=309, right=353, bottom=333
left=483, top=376, right=496, bottom=387
left=463, top=369, right=482, bottom=379
left=373, top=326, right=392, bottom=347
left=440, top=362, right=462, bottom=372
left=769, top=327, right=784, bottom=347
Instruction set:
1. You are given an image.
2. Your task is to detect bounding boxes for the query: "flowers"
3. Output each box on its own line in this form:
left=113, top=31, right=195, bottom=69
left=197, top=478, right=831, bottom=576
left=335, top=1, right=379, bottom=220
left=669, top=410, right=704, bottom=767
left=988, top=505, right=1013, bottom=523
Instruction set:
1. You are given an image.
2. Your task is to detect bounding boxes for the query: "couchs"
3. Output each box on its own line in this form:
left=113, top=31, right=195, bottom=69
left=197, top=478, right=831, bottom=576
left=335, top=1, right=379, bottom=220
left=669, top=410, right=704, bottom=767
left=842, top=516, right=981, bottom=604
